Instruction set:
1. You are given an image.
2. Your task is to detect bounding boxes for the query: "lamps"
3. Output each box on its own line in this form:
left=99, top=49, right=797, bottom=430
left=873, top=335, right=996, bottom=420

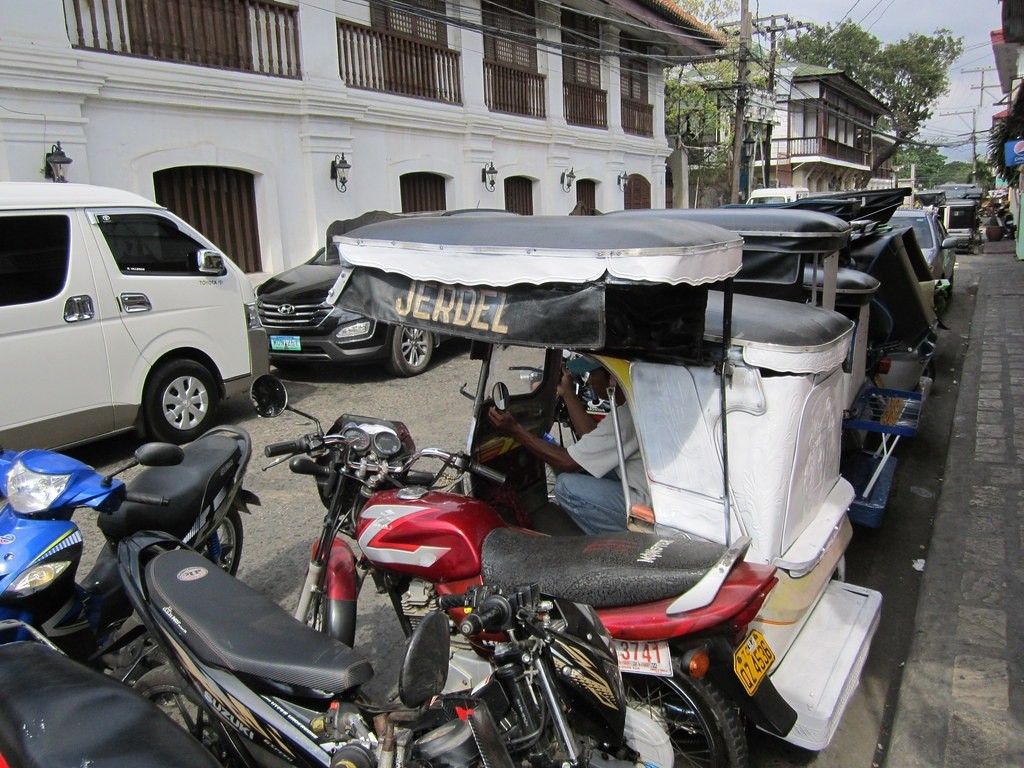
left=618, top=171, right=629, bottom=192
left=45, top=140, right=75, bottom=183
left=332, top=151, right=351, bottom=194
left=561, top=166, right=575, bottom=193
left=482, top=161, right=499, bottom=193
left=743, top=133, right=755, bottom=162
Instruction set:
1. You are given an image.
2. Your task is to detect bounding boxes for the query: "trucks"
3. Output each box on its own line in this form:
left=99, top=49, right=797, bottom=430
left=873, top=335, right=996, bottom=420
left=745, top=187, right=809, bottom=207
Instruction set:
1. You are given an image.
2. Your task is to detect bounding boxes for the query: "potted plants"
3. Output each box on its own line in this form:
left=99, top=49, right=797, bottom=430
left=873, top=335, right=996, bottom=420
left=980, top=201, right=1010, bottom=243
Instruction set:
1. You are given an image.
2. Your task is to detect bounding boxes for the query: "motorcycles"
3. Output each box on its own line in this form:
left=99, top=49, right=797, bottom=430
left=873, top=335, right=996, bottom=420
left=0, top=187, right=1020, bottom=767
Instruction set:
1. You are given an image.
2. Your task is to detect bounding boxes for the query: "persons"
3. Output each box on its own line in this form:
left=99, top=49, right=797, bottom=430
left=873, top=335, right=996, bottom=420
left=489, top=357, right=653, bottom=536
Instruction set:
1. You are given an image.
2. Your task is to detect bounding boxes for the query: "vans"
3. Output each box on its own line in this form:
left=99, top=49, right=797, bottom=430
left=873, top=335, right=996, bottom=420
left=0, top=180, right=280, bottom=454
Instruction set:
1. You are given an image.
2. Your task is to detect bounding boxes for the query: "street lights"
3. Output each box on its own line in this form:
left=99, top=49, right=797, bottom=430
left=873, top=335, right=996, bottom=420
left=742, top=133, right=756, bottom=201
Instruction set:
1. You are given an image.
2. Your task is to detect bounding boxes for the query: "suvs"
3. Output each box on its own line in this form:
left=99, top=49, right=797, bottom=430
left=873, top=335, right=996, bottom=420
left=252, top=209, right=518, bottom=380
